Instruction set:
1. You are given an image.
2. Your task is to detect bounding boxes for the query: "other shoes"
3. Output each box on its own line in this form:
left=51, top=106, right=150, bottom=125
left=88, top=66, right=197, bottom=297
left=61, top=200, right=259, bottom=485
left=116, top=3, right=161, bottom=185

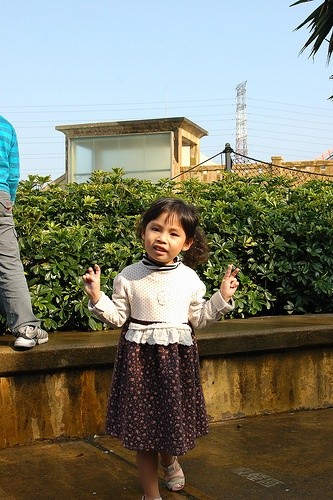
left=161, top=452, right=186, bottom=494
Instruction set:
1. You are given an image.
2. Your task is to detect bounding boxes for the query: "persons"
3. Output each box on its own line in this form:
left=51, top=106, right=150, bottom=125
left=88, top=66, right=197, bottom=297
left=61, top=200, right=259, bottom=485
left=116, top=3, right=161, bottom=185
left=76, top=193, right=244, bottom=500
left=0, top=110, right=54, bottom=349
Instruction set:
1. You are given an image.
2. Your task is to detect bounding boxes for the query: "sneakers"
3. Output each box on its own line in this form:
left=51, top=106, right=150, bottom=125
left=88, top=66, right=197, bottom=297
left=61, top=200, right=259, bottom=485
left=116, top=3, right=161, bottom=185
left=12, top=322, right=50, bottom=349
